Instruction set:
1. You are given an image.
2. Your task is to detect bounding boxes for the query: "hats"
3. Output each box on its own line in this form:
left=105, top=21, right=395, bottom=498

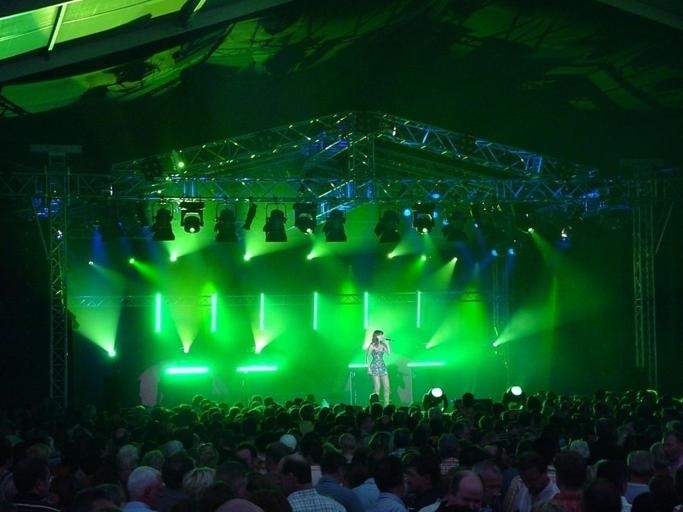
left=560, top=438, right=590, bottom=459
left=279, top=434, right=297, bottom=451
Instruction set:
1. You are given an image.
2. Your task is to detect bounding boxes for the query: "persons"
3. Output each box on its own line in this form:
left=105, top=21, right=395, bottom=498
left=366, top=329, right=391, bottom=404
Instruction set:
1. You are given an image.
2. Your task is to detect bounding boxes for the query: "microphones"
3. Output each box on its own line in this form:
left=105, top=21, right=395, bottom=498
left=380, top=337, right=391, bottom=341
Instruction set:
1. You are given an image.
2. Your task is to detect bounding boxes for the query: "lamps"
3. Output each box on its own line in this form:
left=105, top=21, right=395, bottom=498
left=146, top=198, right=469, bottom=246
